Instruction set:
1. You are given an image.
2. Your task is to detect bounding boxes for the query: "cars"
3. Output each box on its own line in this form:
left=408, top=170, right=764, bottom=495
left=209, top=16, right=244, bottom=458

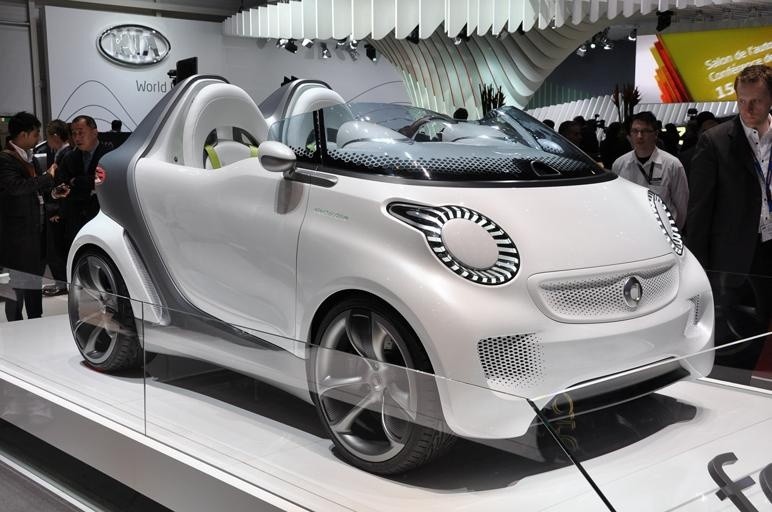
left=30, top=140, right=49, bottom=174
left=66, top=73, right=717, bottom=474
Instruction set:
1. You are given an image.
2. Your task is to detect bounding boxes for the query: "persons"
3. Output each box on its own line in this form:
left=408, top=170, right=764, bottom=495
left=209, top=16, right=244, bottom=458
left=541, top=65, right=772, bottom=384
left=0, top=111, right=122, bottom=321
